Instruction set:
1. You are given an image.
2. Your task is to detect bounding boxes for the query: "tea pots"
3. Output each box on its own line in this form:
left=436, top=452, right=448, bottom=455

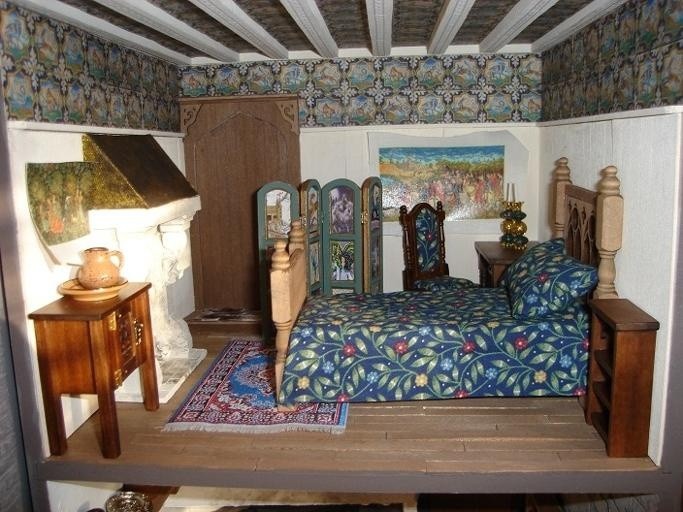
left=76, top=247, right=126, bottom=287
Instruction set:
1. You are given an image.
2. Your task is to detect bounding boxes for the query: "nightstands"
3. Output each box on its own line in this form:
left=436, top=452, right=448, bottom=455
left=475, top=241, right=540, bottom=288
left=584, top=299, right=661, bottom=456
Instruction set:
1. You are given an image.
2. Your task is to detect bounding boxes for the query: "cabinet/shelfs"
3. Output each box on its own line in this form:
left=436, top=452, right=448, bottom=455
left=175, top=94, right=301, bottom=351
left=28, top=280, right=160, bottom=457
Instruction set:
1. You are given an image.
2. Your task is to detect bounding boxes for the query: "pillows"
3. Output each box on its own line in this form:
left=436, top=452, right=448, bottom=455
left=498, top=237, right=597, bottom=320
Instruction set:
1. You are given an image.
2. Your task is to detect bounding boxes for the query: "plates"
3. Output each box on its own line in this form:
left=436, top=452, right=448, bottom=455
left=58, top=276, right=129, bottom=301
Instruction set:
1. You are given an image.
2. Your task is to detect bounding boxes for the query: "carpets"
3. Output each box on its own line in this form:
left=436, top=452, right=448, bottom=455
left=161, top=336, right=348, bottom=435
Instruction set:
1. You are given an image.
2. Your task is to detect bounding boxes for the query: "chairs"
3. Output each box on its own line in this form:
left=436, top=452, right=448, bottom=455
left=398, top=201, right=482, bottom=292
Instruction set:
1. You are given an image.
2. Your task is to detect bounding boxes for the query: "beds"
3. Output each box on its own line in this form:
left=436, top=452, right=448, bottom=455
left=269, top=157, right=622, bottom=411
